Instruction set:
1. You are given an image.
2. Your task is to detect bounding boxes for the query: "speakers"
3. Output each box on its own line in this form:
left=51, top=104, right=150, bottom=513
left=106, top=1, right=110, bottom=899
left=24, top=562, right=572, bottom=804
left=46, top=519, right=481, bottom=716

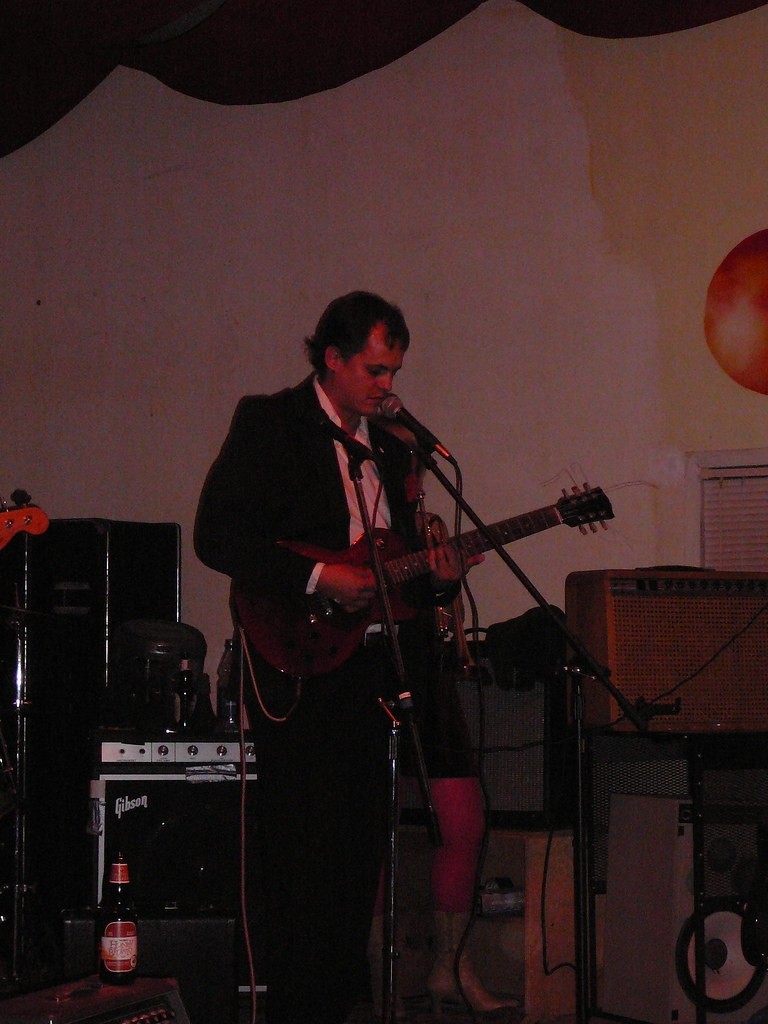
left=391, top=563, right=768, bottom=1024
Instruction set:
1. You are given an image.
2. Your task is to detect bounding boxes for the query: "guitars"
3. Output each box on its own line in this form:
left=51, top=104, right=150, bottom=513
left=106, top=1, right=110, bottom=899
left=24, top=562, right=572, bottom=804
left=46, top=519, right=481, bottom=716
left=227, top=480, right=618, bottom=686
left=0, top=487, right=51, bottom=553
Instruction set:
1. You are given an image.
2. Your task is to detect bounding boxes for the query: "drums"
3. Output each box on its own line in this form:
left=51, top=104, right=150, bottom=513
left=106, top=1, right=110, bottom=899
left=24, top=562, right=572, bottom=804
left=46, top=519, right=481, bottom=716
left=116, top=618, right=208, bottom=715
left=216, top=638, right=235, bottom=685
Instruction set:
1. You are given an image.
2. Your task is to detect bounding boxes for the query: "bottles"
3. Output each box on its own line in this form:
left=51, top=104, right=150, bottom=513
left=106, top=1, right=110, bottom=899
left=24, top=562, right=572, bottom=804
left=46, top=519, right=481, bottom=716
left=96, top=850, right=139, bottom=985
left=216, top=639, right=240, bottom=731
left=172, top=651, right=198, bottom=725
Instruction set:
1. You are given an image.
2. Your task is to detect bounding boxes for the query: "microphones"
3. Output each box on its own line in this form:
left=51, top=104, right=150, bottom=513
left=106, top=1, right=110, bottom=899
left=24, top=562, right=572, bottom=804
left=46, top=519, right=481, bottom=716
left=379, top=393, right=458, bottom=467
left=308, top=410, right=380, bottom=464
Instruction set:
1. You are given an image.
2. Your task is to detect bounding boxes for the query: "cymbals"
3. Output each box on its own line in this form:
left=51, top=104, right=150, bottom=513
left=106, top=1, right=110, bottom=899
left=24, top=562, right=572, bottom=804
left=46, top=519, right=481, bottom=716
left=1, top=603, right=69, bottom=632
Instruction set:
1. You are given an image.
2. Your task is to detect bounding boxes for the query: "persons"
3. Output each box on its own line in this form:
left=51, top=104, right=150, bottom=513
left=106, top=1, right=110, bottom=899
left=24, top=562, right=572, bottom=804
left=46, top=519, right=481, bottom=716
left=368, top=415, right=519, bottom=1021
left=192, top=292, right=483, bottom=1024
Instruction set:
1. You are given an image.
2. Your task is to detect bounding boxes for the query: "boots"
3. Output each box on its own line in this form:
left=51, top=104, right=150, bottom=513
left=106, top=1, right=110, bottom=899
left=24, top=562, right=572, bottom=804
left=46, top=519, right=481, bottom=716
left=366, top=914, right=408, bottom=1023
left=425, top=911, right=521, bottom=1016
